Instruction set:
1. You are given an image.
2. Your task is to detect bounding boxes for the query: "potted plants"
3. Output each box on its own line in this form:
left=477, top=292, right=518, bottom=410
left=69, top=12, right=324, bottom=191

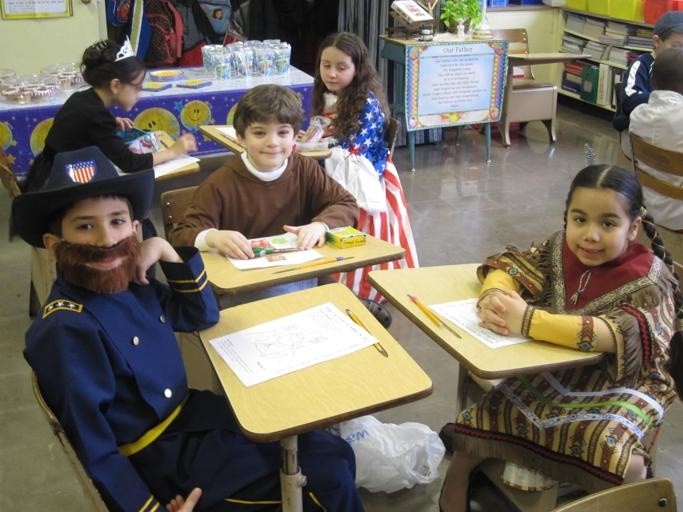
left=440, top=0, right=482, bottom=36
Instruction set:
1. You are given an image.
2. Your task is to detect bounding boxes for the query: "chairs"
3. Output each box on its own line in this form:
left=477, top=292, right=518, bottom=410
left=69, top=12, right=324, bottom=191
left=625, top=132, right=682, bottom=333
left=158, top=186, right=197, bottom=240
left=385, top=118, right=400, bottom=154
left=490, top=29, right=556, bottom=145
left=21, top=334, right=110, bottom=511
left=550, top=477, right=677, bottom=511
left=0, top=146, right=55, bottom=317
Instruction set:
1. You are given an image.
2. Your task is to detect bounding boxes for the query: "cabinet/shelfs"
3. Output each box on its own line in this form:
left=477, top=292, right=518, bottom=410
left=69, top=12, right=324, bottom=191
left=562, top=7, right=660, bottom=114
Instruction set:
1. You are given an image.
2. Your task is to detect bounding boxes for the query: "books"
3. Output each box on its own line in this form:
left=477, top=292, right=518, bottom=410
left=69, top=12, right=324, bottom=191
left=559, top=12, right=655, bottom=116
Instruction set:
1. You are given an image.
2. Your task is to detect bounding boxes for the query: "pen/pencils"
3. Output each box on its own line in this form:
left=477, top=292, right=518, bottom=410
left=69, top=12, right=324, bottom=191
left=346, top=310, right=388, bottom=357
left=272, top=257, right=344, bottom=275
left=407, top=294, right=462, bottom=339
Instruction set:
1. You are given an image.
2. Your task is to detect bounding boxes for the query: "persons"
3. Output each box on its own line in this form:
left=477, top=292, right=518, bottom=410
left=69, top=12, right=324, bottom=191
left=613, top=12, right=682, bottom=158
left=628, top=49, right=683, bottom=231
left=433, top=164, right=682, bottom=511
left=26, top=33, right=197, bottom=279
left=11, top=144, right=370, bottom=511
left=310, top=30, right=424, bottom=306
left=165, top=486, right=202, bottom=511
left=170, top=84, right=393, bottom=333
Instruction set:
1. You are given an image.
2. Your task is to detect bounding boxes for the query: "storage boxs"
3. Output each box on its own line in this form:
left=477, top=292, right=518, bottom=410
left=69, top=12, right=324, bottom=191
left=541, top=0, right=682, bottom=25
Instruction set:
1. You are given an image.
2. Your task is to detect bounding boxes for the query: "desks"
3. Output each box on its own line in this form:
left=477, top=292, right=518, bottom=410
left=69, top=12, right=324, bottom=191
left=500, top=51, right=592, bottom=151
left=367, top=261, right=608, bottom=442
left=137, top=128, right=202, bottom=183
left=198, top=224, right=406, bottom=294
left=0, top=60, right=316, bottom=179
left=377, top=31, right=505, bottom=174
left=197, top=123, right=332, bottom=162
left=196, top=284, right=434, bottom=511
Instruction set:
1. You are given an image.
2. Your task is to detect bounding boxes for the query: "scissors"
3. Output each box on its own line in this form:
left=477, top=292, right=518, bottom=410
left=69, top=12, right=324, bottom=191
left=253, top=248, right=298, bottom=257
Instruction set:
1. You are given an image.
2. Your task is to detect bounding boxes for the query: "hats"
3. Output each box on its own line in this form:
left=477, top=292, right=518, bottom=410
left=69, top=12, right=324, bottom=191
left=12, top=145, right=156, bottom=242
left=653, top=10, right=683, bottom=36
